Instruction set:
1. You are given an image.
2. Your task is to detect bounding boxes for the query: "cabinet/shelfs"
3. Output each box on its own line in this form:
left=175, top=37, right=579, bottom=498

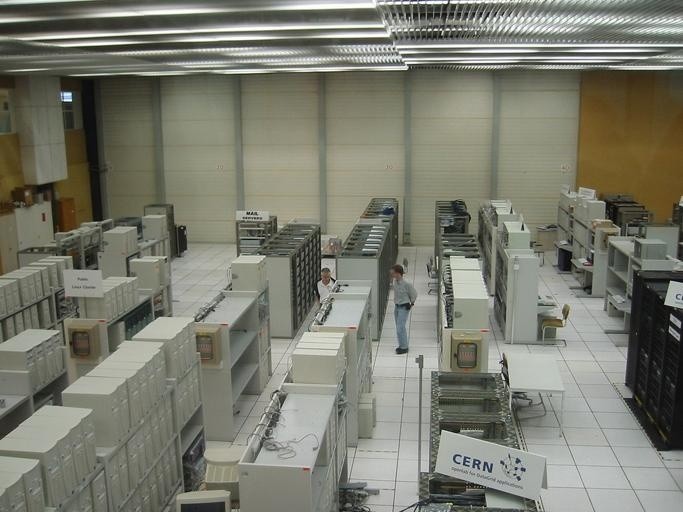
left=429, top=199, right=539, bottom=512
left=238, top=278, right=374, bottom=511
left=1, top=198, right=207, bottom=512
left=555, top=188, right=682, bottom=451
left=192, top=254, right=271, bottom=443
left=334, top=197, right=398, bottom=341
left=251, top=222, right=321, bottom=338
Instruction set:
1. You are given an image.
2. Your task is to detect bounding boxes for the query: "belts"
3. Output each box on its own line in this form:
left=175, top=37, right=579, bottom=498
left=394, top=302, right=408, bottom=307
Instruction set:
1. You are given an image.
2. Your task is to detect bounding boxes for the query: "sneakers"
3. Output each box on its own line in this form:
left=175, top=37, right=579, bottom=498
left=396, top=347, right=408, bottom=354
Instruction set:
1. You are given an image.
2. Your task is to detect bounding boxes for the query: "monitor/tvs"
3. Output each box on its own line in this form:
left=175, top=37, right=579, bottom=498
left=203, top=446, right=255, bottom=500
left=175, top=489, right=232, bottom=512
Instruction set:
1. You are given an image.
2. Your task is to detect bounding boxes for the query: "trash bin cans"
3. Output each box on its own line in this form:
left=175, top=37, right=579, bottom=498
left=178, top=226, right=187, bottom=252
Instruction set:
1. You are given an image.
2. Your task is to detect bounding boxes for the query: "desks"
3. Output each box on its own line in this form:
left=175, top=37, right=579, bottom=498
left=506, top=352, right=566, bottom=438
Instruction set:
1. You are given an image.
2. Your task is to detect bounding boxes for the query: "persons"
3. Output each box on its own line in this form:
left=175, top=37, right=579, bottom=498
left=389, top=264, right=418, bottom=354
left=314, top=267, right=337, bottom=307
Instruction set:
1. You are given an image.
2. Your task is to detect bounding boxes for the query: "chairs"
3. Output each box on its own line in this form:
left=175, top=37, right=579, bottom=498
left=541, top=304, right=570, bottom=348
